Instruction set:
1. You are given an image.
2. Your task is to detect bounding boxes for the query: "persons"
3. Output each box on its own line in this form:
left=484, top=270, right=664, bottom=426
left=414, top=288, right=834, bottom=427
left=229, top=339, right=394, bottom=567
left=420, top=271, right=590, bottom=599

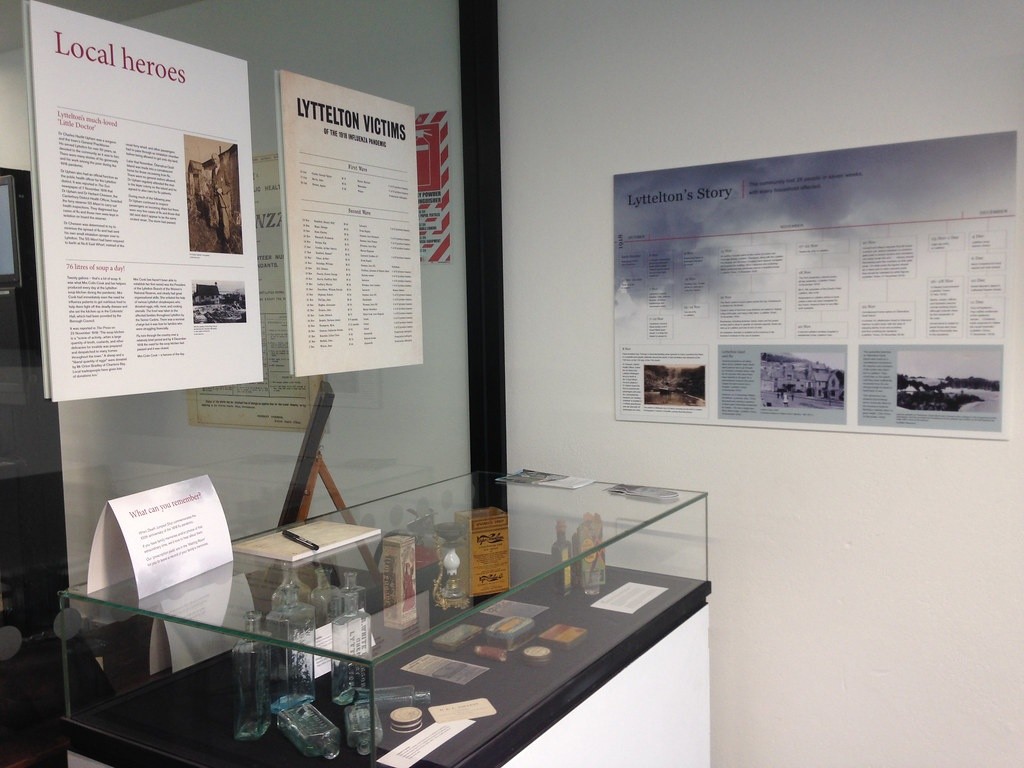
left=207, top=152, right=231, bottom=242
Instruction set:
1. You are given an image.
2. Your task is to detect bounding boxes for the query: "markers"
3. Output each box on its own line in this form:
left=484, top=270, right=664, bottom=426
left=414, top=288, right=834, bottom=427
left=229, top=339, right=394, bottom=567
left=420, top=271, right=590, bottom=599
left=282, top=529, right=319, bottom=551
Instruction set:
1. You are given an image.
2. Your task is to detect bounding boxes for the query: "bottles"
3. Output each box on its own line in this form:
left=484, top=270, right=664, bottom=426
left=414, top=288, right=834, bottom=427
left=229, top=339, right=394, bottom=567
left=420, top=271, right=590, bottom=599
left=551, top=519, right=572, bottom=595
left=354, top=684, right=431, bottom=708
left=228, top=561, right=384, bottom=758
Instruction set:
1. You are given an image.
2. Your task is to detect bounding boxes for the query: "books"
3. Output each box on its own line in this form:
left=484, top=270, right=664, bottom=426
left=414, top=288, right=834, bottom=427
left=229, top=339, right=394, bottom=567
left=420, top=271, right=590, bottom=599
left=233, top=517, right=381, bottom=561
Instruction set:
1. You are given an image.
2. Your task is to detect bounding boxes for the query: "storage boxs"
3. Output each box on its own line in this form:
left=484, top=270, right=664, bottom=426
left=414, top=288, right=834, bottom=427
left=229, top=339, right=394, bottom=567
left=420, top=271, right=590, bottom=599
left=454, top=507, right=509, bottom=598
left=383, top=536, right=417, bottom=631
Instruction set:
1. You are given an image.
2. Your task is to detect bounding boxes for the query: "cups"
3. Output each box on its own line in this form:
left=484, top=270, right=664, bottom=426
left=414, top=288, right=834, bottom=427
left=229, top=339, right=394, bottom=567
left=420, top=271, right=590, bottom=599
left=582, top=568, right=601, bottom=595
